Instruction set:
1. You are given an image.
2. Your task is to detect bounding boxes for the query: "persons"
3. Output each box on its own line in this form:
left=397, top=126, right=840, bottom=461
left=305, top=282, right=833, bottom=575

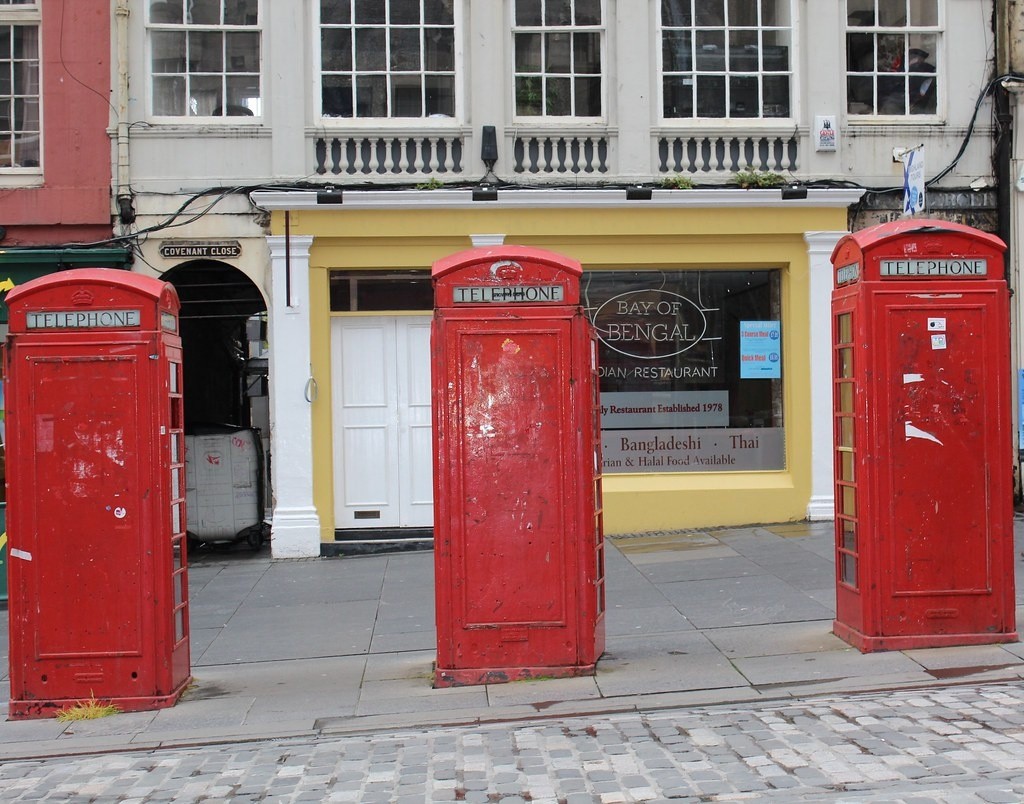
left=883, top=47, right=938, bottom=115
